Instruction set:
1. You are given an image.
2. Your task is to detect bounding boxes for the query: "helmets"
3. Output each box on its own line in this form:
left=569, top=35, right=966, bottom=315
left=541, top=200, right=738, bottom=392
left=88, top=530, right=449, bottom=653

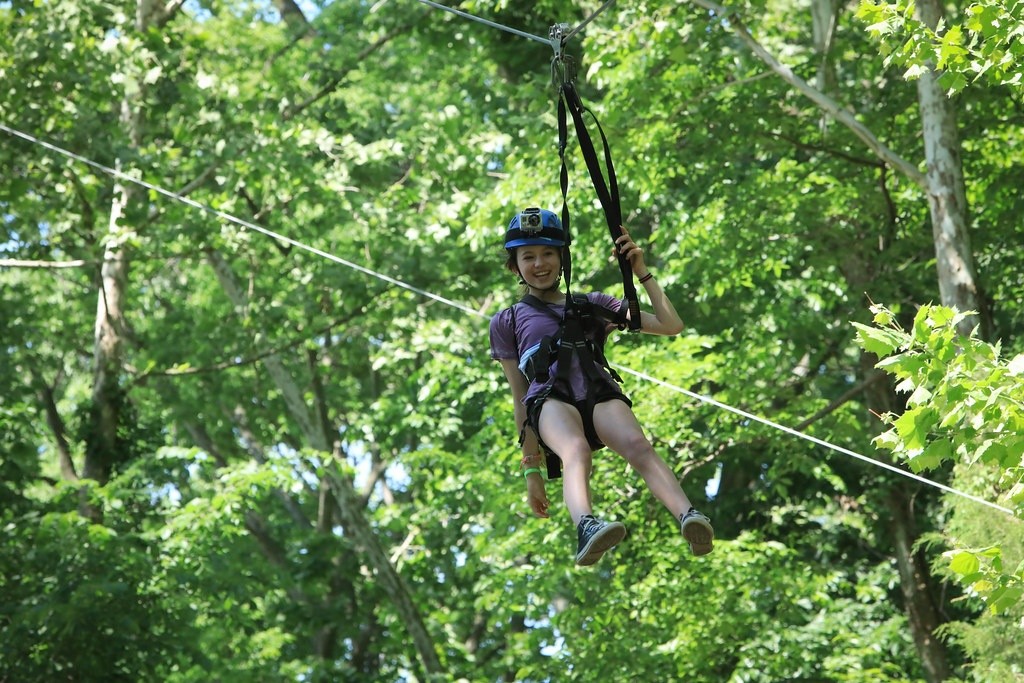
left=504, top=208, right=571, bottom=249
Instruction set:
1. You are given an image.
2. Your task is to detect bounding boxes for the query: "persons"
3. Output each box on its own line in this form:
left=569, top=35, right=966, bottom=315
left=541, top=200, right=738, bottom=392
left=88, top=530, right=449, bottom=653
left=490, top=208, right=713, bottom=565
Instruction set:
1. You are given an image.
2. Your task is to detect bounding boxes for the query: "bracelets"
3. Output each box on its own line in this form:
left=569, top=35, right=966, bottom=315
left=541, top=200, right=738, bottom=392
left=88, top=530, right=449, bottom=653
left=639, top=273, right=652, bottom=283
left=524, top=468, right=543, bottom=478
left=518, top=454, right=541, bottom=469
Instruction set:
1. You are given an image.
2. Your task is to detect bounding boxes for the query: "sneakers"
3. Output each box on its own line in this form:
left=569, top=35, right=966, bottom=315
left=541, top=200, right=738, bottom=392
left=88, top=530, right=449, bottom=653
left=679, top=507, right=713, bottom=556
left=576, top=514, right=626, bottom=565
